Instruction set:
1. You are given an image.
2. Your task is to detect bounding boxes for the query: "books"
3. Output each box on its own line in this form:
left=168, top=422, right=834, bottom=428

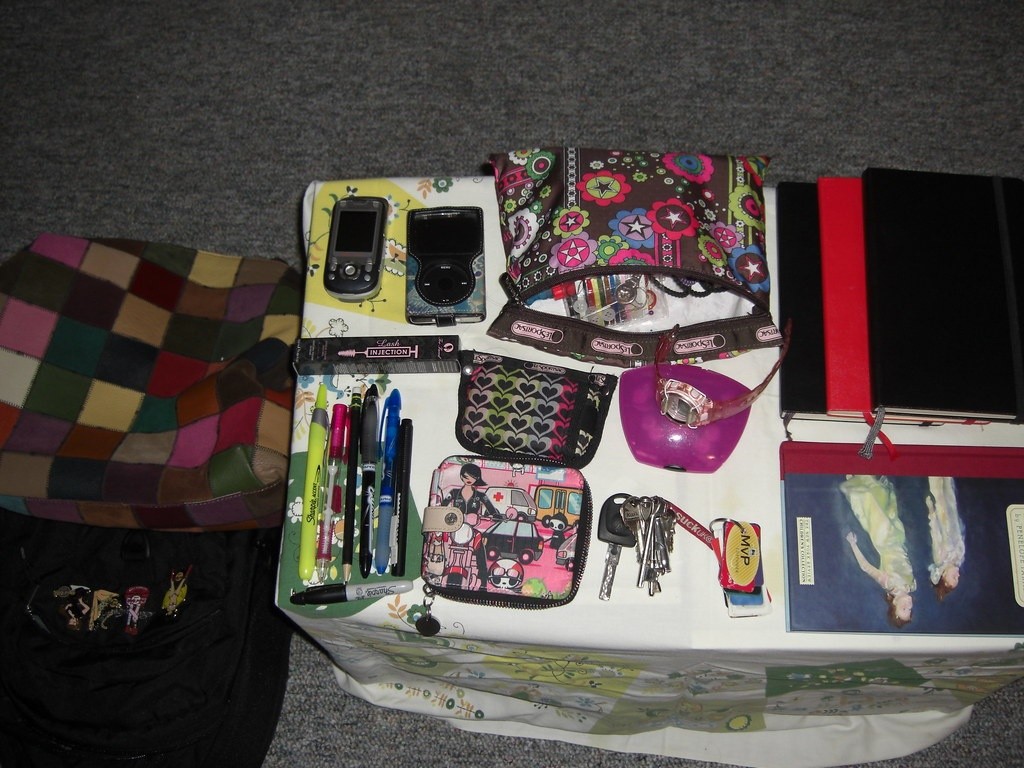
left=779, top=440, right=1024, bottom=637
left=776, top=168, right=1024, bottom=426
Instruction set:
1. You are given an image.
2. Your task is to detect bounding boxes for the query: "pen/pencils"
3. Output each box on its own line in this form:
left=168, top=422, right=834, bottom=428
left=289, top=578, right=414, bottom=606
left=374, top=388, right=403, bottom=574
left=389, top=418, right=413, bottom=576
left=299, top=383, right=330, bottom=581
left=360, top=383, right=380, bottom=578
left=317, top=403, right=349, bottom=584
left=342, top=386, right=361, bottom=586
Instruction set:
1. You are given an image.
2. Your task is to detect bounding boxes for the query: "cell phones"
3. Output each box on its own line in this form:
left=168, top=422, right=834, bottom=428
left=322, top=196, right=388, bottom=303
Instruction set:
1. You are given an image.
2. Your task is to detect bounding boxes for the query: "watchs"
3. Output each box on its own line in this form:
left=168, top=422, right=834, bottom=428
left=654, top=317, right=791, bottom=430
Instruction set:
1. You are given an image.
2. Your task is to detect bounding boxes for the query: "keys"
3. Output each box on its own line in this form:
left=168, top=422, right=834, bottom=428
left=620, top=496, right=677, bottom=595
left=616, top=273, right=642, bottom=304
left=598, top=493, right=636, bottom=601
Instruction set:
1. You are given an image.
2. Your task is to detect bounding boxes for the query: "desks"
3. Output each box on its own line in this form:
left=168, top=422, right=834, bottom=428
left=275, top=176, right=1024, bottom=768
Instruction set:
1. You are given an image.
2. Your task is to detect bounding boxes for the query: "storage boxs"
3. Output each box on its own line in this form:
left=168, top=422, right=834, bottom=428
left=294, top=337, right=462, bottom=376
